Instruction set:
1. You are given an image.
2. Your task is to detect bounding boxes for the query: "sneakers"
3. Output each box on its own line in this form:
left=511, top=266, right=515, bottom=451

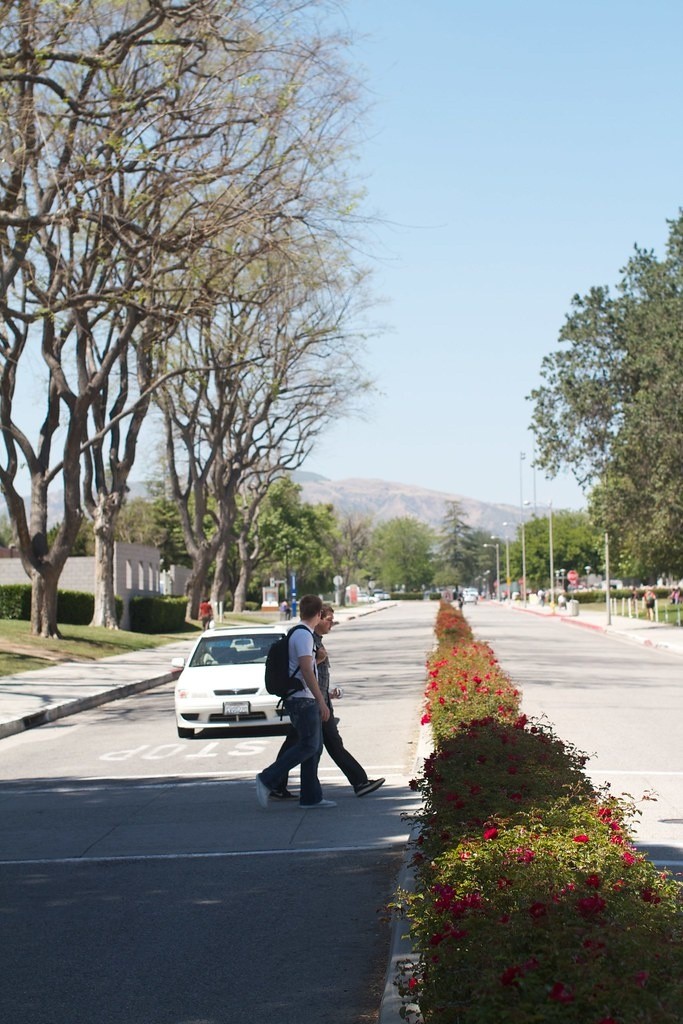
left=299, top=799, right=337, bottom=809
left=256, top=774, right=271, bottom=808
left=269, top=788, right=300, bottom=801
left=354, top=778, right=386, bottom=797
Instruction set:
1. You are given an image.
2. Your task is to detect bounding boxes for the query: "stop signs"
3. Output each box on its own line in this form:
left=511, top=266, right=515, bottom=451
left=567, top=570, right=578, bottom=582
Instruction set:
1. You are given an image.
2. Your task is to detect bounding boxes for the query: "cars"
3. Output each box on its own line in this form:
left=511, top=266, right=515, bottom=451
left=461, top=588, right=479, bottom=604
left=170, top=624, right=297, bottom=738
left=358, top=588, right=392, bottom=604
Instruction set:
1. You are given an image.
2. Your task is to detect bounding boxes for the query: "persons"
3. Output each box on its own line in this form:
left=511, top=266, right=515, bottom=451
left=644, top=587, right=656, bottom=623
left=538, top=587, right=550, bottom=607
left=457, top=594, right=464, bottom=611
left=558, top=593, right=567, bottom=609
left=632, top=587, right=637, bottom=606
left=255, top=595, right=337, bottom=809
left=198, top=597, right=214, bottom=631
left=491, top=592, right=496, bottom=600
left=268, top=604, right=386, bottom=802
left=367, top=588, right=371, bottom=602
left=501, top=591, right=506, bottom=601
left=481, top=590, right=486, bottom=598
left=668, top=587, right=681, bottom=605
left=280, top=599, right=292, bottom=620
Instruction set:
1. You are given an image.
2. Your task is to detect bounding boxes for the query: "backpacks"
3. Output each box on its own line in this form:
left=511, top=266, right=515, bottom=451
left=265, top=624, right=315, bottom=720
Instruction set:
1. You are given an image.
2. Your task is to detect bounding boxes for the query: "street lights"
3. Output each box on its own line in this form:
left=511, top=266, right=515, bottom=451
left=483, top=543, right=499, bottom=605
left=522, top=500, right=556, bottom=607
left=490, top=535, right=511, bottom=601
left=502, top=521, right=526, bottom=604
